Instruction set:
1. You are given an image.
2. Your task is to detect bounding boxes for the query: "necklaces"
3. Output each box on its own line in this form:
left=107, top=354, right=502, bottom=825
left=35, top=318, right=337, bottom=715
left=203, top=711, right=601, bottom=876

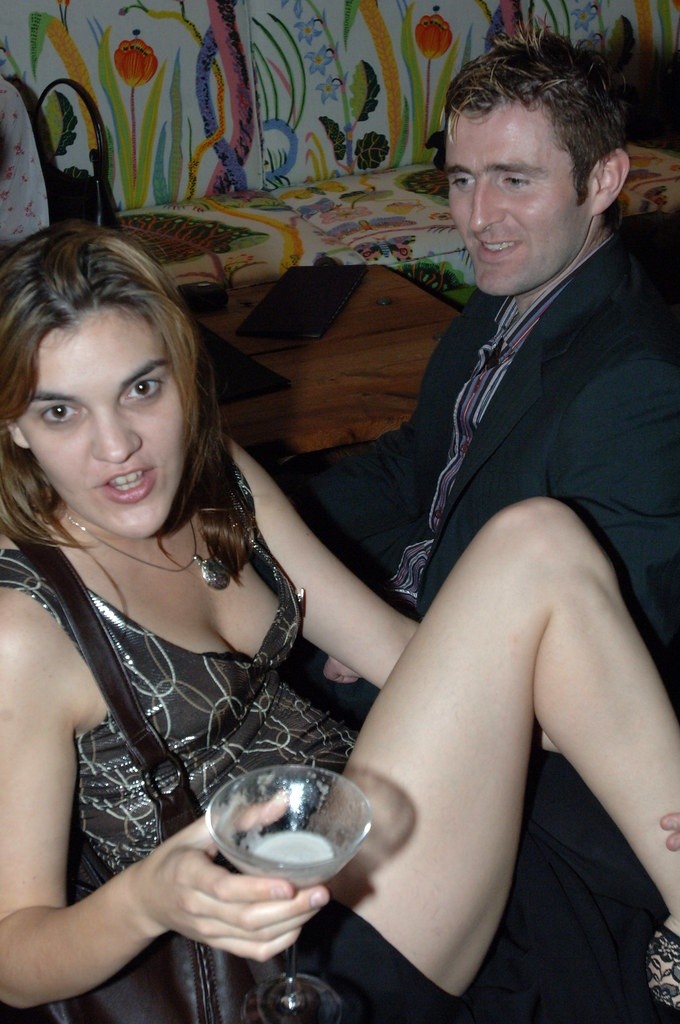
left=68, top=516, right=231, bottom=590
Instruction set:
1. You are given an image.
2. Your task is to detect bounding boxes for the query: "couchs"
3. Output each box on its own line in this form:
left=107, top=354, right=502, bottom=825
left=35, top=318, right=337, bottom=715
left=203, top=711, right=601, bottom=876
left=0, top=0, right=680, bottom=309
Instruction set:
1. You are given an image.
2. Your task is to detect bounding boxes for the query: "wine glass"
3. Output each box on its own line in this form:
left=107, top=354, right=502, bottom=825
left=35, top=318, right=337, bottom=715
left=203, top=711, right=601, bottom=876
left=205, top=765, right=372, bottom=1024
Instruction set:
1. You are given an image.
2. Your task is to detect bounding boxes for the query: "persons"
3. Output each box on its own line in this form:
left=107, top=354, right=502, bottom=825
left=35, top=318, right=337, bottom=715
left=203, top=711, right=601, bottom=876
left=268, top=18, right=680, bottom=1023
left=0, top=222, right=680, bottom=1024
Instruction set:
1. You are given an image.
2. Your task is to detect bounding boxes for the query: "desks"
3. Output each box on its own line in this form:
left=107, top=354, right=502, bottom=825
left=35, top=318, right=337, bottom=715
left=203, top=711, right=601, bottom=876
left=169, top=262, right=458, bottom=474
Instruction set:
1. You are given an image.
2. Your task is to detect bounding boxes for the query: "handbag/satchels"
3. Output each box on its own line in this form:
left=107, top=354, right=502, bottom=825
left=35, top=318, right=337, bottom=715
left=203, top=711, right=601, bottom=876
left=0, top=537, right=319, bottom=1024
left=32, top=78, right=120, bottom=232
